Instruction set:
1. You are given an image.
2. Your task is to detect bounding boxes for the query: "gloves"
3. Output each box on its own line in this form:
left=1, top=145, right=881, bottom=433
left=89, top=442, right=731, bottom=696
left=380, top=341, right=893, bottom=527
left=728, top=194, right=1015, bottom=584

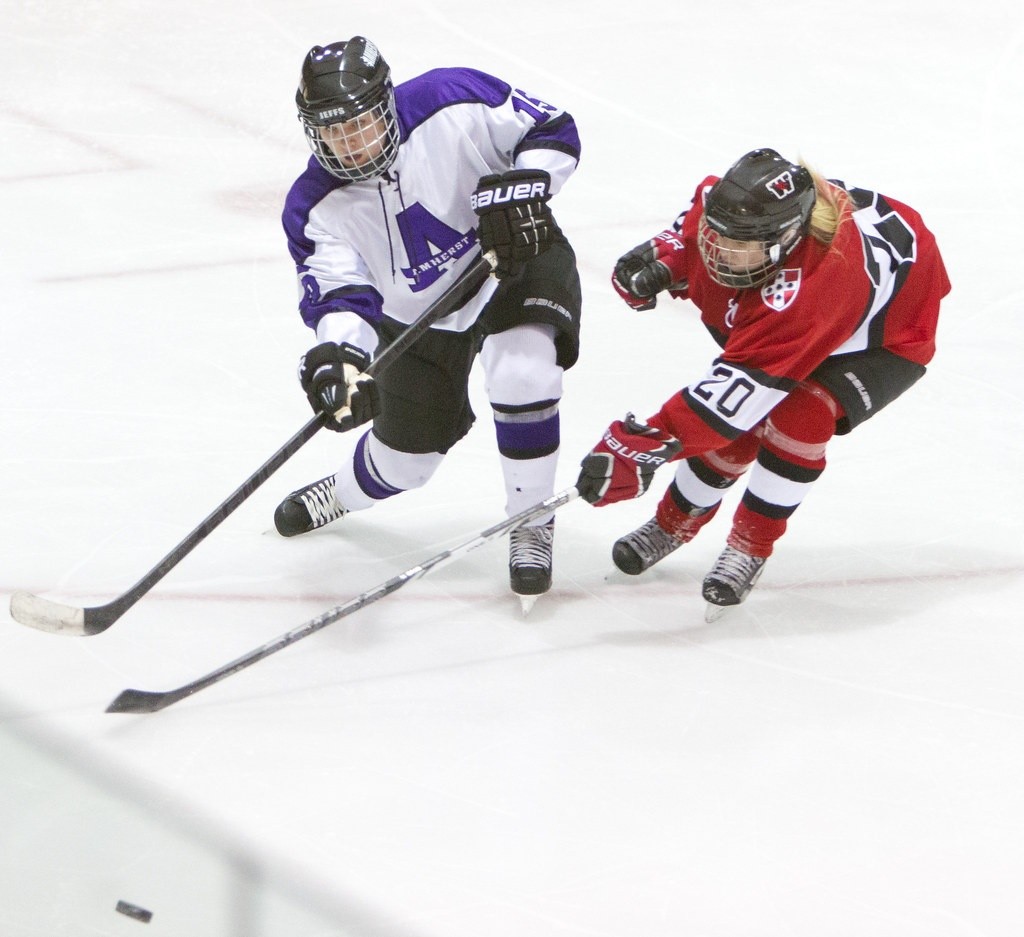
left=470, top=168, right=554, bottom=280
left=297, top=339, right=383, bottom=433
left=612, top=228, right=689, bottom=311
left=576, top=411, right=684, bottom=507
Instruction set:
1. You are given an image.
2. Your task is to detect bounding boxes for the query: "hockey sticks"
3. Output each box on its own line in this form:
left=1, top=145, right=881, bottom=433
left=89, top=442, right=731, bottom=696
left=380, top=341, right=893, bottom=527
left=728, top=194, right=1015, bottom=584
left=101, top=478, right=590, bottom=713
left=11, top=223, right=516, bottom=637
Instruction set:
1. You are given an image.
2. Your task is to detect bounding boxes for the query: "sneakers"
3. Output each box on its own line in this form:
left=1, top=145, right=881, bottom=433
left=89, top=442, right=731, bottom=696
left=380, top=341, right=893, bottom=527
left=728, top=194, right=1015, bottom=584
left=701, top=543, right=768, bottom=622
left=613, top=515, right=685, bottom=575
left=508, top=514, right=555, bottom=617
left=274, top=470, right=350, bottom=536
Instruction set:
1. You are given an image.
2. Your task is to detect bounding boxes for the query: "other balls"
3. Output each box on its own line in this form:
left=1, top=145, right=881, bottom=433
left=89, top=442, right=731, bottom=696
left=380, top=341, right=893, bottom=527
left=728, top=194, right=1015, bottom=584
left=117, top=898, right=154, bottom=926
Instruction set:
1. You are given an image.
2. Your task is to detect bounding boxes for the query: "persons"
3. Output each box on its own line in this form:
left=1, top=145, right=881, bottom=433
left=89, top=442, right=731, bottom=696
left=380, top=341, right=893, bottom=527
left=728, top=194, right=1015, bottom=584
left=261, top=36, right=583, bottom=620
left=577, top=148, right=954, bottom=625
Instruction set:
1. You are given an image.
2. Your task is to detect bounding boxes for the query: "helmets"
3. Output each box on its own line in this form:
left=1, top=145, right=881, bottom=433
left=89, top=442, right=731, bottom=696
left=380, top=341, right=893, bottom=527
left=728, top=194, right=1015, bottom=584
left=696, top=147, right=816, bottom=288
left=295, top=34, right=401, bottom=183
